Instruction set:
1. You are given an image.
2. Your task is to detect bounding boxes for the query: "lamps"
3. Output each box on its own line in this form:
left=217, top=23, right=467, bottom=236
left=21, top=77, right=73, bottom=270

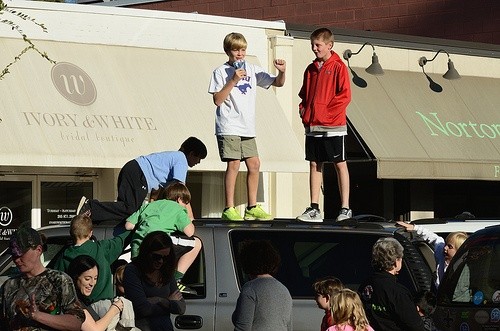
left=343, top=42, right=384, bottom=75
left=419, top=49, right=461, bottom=80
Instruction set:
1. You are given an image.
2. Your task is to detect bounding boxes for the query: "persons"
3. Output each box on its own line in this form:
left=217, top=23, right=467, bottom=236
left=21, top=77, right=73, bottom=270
left=0, top=227, right=86, bottom=331
left=53, top=213, right=133, bottom=313
left=358, top=237, right=428, bottom=331
left=209, top=32, right=287, bottom=222
left=67, top=255, right=125, bottom=331
left=124, top=231, right=186, bottom=331
left=232, top=242, right=293, bottom=331
left=313, top=275, right=344, bottom=331
left=76, top=136, right=208, bottom=224
left=124, top=183, right=201, bottom=295
left=325, top=288, right=375, bottom=331
left=114, top=266, right=126, bottom=294
left=396, top=220, right=472, bottom=294
left=295, top=28, right=352, bottom=223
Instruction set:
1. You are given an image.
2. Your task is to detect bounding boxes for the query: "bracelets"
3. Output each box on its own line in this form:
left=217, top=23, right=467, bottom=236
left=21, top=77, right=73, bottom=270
left=112, top=304, right=121, bottom=311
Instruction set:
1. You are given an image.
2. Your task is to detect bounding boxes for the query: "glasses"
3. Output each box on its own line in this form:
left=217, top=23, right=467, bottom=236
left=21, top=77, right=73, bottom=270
left=154, top=254, right=168, bottom=262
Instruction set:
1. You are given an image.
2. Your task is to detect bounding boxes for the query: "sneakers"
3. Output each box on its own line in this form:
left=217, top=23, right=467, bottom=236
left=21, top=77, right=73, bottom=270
left=76, top=196, right=91, bottom=218
left=222, top=207, right=243, bottom=221
left=336, top=208, right=352, bottom=222
left=296, top=207, right=323, bottom=222
left=244, top=204, right=274, bottom=221
left=176, top=282, right=192, bottom=293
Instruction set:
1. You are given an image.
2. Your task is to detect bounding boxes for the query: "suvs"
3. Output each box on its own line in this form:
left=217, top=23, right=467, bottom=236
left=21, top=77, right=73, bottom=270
left=351, top=211, right=500, bottom=331
left=47, top=215, right=439, bottom=331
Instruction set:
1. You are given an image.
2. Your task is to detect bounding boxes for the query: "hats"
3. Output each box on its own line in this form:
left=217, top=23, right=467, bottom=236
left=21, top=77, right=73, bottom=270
left=8, top=229, right=40, bottom=257
